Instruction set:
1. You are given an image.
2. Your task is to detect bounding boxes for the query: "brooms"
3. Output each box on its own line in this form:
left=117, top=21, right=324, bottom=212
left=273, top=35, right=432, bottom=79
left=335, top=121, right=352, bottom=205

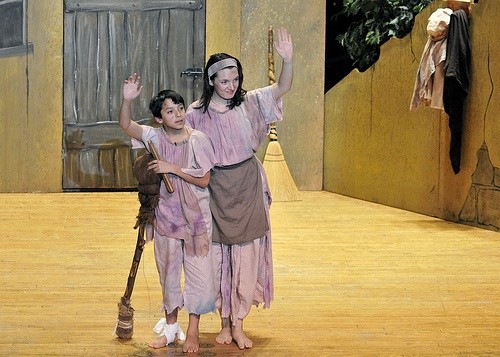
left=256, top=24, right=302, bottom=201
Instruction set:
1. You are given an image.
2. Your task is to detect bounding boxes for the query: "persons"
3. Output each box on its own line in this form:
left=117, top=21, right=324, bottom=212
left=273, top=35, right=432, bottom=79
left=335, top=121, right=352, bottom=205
left=118, top=71, right=219, bottom=354
left=183, top=25, right=296, bottom=353
